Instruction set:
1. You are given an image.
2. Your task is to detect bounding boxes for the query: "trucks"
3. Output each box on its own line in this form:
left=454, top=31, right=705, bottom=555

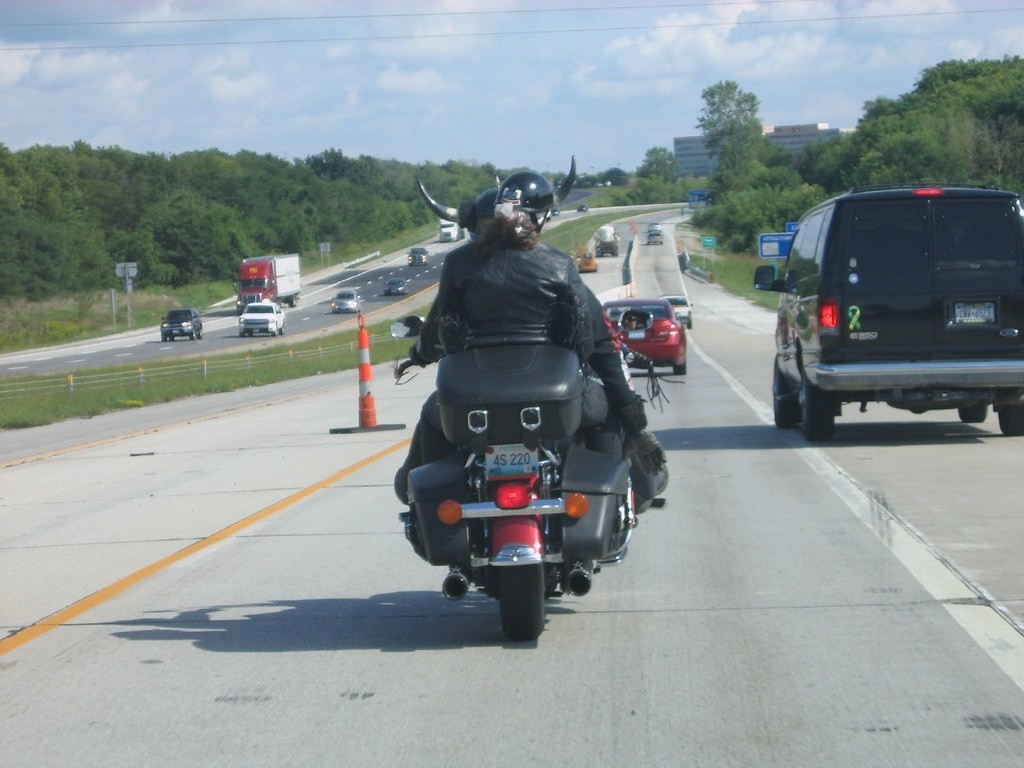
left=238, top=252, right=301, bottom=311
left=594, top=224, right=619, bottom=258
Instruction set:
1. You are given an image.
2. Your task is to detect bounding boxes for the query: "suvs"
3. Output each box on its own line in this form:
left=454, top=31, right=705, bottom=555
left=239, top=300, right=286, bottom=339
left=159, top=307, right=203, bottom=340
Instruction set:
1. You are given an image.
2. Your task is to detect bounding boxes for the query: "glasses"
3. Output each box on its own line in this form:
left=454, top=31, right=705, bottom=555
left=624, top=316, right=637, bottom=321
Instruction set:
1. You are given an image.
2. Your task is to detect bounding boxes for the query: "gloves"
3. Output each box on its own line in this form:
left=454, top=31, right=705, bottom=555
left=410, top=346, right=428, bottom=368
left=631, top=426, right=668, bottom=477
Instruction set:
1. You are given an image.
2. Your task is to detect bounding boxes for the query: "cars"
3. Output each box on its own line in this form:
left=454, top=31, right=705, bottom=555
left=659, top=295, right=695, bottom=331
left=601, top=296, right=685, bottom=375
left=647, top=223, right=661, bottom=233
left=407, top=247, right=429, bottom=265
left=578, top=251, right=598, bottom=274
left=330, top=289, right=361, bottom=313
left=646, top=230, right=663, bottom=245
left=384, top=277, right=409, bottom=295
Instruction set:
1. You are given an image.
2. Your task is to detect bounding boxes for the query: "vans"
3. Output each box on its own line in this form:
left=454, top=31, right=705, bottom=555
left=436, top=220, right=467, bottom=243
left=754, top=182, right=1023, bottom=442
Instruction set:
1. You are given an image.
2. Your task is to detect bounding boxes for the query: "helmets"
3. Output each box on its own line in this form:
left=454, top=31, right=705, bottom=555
left=417, top=155, right=578, bottom=240
left=495, top=171, right=555, bottom=212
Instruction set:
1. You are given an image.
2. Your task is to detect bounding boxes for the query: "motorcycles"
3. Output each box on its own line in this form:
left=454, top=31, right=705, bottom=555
left=388, top=310, right=666, bottom=642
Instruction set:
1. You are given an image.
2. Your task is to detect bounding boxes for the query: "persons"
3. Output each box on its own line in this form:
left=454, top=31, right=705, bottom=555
left=623, top=311, right=641, bottom=329
left=397, top=175, right=669, bottom=513
